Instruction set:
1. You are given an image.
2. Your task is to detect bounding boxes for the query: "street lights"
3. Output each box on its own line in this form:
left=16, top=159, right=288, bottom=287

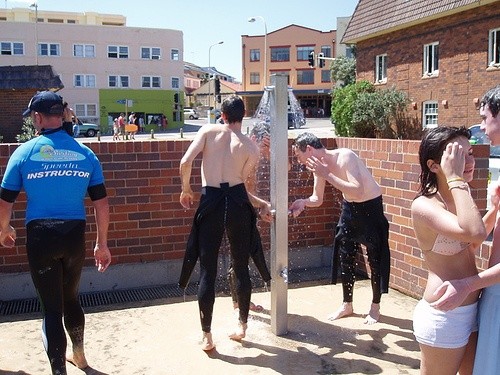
left=247, top=15, right=267, bottom=88
left=208, top=40, right=224, bottom=67
left=27, top=2, right=40, bottom=66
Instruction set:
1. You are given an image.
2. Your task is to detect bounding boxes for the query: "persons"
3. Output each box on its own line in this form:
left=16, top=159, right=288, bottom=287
left=62, top=101, right=78, bottom=136
left=112, top=112, right=137, bottom=141
left=303, top=105, right=329, bottom=117
left=0, top=90, right=111, bottom=375
left=228, top=116, right=291, bottom=311
left=150, top=115, right=168, bottom=131
left=411, top=125, right=500, bottom=373
left=179, top=95, right=261, bottom=350
left=287, top=132, right=390, bottom=326
left=426, top=87, right=500, bottom=375
left=138, top=115, right=145, bottom=131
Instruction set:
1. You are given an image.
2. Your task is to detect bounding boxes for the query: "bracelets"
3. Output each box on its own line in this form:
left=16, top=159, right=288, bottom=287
left=446, top=184, right=469, bottom=190
left=446, top=178, right=465, bottom=183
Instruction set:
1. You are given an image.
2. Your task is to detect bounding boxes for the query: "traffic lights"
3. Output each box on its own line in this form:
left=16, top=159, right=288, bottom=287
left=215, top=78, right=220, bottom=94
left=218, top=95, right=221, bottom=104
left=319, top=52, right=326, bottom=68
left=308, top=52, right=315, bottom=68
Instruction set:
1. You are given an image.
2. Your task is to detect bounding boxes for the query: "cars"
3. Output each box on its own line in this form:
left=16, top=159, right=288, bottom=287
left=184, top=108, right=200, bottom=121
left=72, top=116, right=100, bottom=137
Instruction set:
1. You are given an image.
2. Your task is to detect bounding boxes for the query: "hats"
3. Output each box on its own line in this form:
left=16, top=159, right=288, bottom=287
left=131, top=112, right=134, bottom=114
left=22, top=91, right=63, bottom=117
left=115, top=118, right=118, bottom=122
